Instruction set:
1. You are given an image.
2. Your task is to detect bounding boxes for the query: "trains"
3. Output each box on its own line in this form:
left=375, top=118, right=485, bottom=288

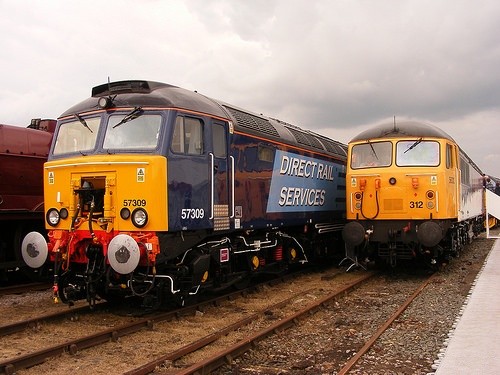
left=20, top=76, right=348, bottom=316
left=340, top=115, right=500, bottom=276
left=0, top=119, right=58, bottom=281
